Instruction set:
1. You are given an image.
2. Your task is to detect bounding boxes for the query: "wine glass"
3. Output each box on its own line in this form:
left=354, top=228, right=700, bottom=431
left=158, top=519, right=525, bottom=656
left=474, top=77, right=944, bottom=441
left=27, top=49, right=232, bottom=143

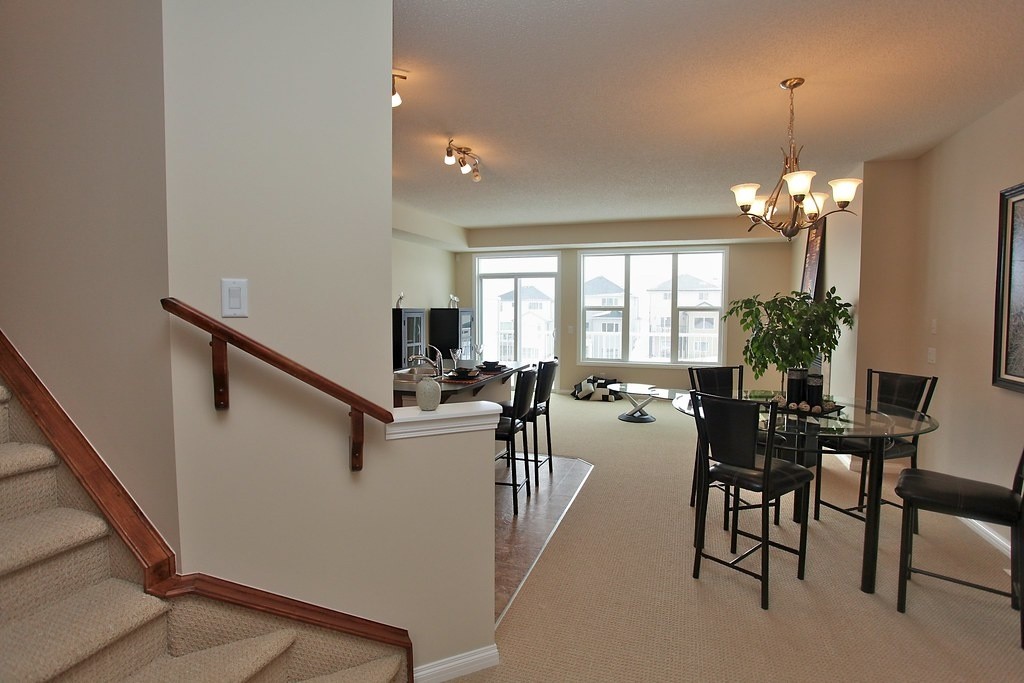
left=450, top=349, right=461, bottom=369
left=472, top=345, right=484, bottom=366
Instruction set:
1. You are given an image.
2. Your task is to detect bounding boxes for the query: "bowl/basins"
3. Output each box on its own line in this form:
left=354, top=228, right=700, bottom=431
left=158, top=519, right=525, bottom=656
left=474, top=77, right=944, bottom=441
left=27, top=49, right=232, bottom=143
left=455, top=368, right=472, bottom=376
left=484, top=360, right=498, bottom=368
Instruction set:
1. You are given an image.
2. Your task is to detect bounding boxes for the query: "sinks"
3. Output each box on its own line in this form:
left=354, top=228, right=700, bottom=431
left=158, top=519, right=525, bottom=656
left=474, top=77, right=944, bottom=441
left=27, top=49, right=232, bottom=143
left=393, top=365, right=454, bottom=381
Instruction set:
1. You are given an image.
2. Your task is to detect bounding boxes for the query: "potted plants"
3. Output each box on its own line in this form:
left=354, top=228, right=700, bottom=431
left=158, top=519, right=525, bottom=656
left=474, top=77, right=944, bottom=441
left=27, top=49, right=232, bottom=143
left=722, top=285, right=856, bottom=409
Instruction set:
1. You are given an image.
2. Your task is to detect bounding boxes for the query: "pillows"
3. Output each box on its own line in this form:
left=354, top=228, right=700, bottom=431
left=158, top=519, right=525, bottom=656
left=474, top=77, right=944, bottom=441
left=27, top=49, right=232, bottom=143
left=574, top=375, right=595, bottom=400
left=589, top=376, right=616, bottom=402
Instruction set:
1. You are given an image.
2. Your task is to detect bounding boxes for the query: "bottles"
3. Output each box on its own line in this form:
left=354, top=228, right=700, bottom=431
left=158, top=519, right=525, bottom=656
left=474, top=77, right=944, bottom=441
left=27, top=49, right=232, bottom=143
left=416, top=378, right=441, bottom=411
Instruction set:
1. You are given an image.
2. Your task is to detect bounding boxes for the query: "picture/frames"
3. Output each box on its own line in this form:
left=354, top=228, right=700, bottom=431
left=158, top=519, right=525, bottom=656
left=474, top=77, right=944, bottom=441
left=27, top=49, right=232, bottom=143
left=991, top=181, right=1024, bottom=395
left=800, top=215, right=826, bottom=303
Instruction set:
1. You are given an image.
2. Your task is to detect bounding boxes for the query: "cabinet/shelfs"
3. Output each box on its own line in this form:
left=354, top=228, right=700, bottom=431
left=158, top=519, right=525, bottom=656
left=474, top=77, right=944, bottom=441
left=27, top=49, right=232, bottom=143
left=429, top=308, right=473, bottom=360
left=392, top=307, right=425, bottom=371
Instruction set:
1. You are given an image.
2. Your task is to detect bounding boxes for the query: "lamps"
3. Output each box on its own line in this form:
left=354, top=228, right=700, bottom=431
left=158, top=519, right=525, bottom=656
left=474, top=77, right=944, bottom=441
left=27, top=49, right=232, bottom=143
left=730, top=78, right=863, bottom=242
left=392, top=74, right=407, bottom=108
left=444, top=139, right=482, bottom=182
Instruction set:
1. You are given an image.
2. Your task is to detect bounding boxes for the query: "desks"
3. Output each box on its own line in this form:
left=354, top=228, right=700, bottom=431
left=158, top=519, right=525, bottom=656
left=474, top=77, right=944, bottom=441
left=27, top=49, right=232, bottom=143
left=669, top=390, right=940, bottom=593
left=393, top=359, right=530, bottom=461
left=607, top=383, right=689, bottom=423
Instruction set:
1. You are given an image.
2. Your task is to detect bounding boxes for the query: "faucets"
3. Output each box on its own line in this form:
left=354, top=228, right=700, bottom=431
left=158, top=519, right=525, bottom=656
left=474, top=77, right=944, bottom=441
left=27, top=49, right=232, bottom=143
left=408, top=343, right=444, bottom=376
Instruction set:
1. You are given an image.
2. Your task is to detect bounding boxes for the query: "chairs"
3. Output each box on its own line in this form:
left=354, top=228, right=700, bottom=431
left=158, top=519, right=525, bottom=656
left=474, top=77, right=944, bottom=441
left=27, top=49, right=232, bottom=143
left=693, top=389, right=814, bottom=610
left=895, top=449, right=1024, bottom=650
left=688, top=365, right=786, bottom=526
left=495, top=356, right=559, bottom=518
left=813, top=366, right=939, bottom=535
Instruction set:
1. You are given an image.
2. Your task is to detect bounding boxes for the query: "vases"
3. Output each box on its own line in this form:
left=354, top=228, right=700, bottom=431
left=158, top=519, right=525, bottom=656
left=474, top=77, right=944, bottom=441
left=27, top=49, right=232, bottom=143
left=416, top=377, right=441, bottom=412
left=807, top=372, right=823, bottom=408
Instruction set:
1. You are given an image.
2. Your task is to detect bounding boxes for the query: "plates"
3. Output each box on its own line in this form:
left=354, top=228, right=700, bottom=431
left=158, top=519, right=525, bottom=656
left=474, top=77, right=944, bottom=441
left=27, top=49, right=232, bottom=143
left=449, top=371, right=479, bottom=380
left=476, top=365, right=506, bottom=371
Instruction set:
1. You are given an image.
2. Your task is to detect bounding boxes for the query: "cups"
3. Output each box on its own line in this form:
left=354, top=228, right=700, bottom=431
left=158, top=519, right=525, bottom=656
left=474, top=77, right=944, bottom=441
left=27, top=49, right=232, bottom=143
left=807, top=374, right=823, bottom=411
